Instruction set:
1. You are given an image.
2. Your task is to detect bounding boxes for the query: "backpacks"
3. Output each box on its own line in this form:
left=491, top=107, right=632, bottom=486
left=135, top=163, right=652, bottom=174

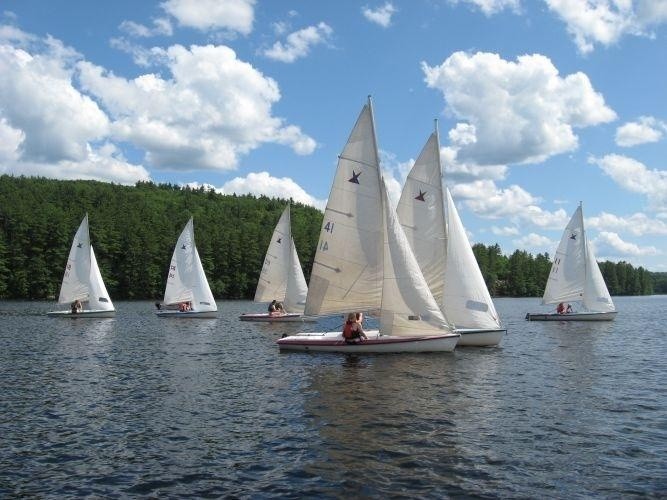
left=342, top=321, right=360, bottom=339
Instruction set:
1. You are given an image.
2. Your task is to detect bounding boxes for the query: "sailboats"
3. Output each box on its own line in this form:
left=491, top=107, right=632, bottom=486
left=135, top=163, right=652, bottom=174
left=47, top=211, right=116, bottom=319
left=157, top=214, right=219, bottom=320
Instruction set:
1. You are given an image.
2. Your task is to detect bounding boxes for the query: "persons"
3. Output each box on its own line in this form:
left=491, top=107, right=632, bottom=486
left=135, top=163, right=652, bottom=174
left=266, top=299, right=281, bottom=316
left=178, top=303, right=191, bottom=312
left=565, top=303, right=573, bottom=314
left=555, top=302, right=564, bottom=314
left=355, top=313, right=362, bottom=323
left=69, top=300, right=83, bottom=313
left=277, top=303, right=286, bottom=314
left=342, top=313, right=367, bottom=345
